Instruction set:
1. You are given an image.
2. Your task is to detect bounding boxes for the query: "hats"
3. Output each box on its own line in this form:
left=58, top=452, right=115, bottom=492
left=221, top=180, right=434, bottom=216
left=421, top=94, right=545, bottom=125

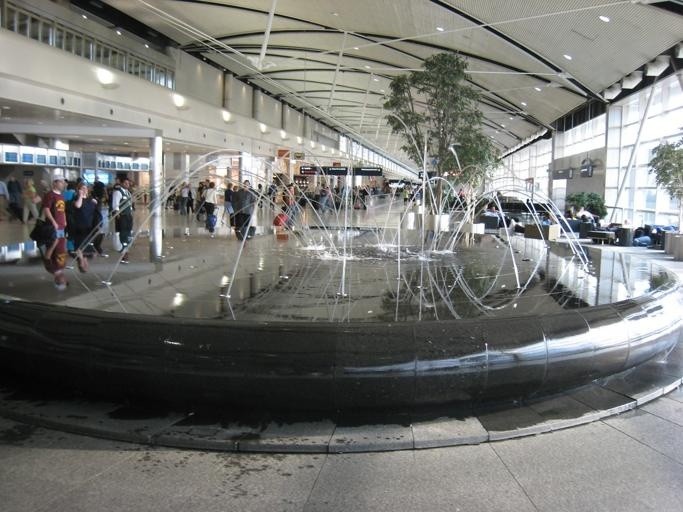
left=51, top=175, right=65, bottom=182
left=53, top=281, right=68, bottom=292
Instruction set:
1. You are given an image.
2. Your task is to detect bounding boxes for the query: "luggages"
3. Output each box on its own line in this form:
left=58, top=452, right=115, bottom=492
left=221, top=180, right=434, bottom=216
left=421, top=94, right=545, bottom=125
left=204, top=207, right=220, bottom=232
left=173, top=199, right=180, bottom=211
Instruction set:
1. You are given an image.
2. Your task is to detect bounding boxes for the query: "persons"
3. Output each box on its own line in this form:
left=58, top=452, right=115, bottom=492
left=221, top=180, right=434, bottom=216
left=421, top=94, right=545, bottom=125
left=43, top=174, right=136, bottom=264
left=283, top=182, right=295, bottom=211
left=2, top=176, right=51, bottom=224
left=117, top=231, right=132, bottom=264
left=542, top=212, right=554, bottom=226
left=578, top=214, right=595, bottom=239
left=564, top=209, right=577, bottom=221
left=169, top=180, right=218, bottom=238
left=457, top=188, right=464, bottom=200
left=221, top=180, right=278, bottom=241
left=320, top=182, right=420, bottom=216
left=67, top=237, right=96, bottom=273
left=576, top=206, right=596, bottom=225
left=480, top=206, right=519, bottom=228
left=36, top=238, right=68, bottom=291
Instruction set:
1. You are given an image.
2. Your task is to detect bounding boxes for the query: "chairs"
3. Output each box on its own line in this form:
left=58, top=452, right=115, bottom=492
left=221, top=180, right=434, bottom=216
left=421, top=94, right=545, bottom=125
left=481, top=204, right=679, bottom=250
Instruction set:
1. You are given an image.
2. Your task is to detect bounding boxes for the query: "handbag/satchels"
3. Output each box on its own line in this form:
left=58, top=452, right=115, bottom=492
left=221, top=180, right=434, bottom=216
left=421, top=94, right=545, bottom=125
left=29, top=218, right=54, bottom=244
left=116, top=188, right=132, bottom=217
